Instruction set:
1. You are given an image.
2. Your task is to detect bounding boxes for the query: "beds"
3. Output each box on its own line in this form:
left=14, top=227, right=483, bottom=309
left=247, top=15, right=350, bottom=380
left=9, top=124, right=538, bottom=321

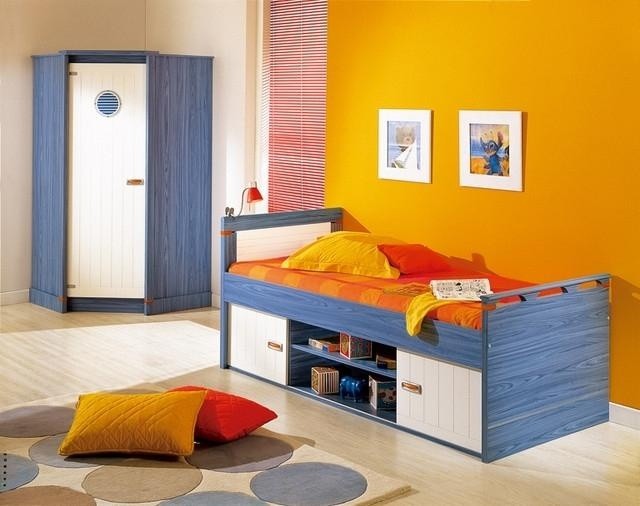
left=220, top=207, right=613, bottom=463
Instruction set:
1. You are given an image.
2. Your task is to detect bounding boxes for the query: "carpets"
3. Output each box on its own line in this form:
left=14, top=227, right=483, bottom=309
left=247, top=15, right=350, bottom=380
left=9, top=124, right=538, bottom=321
left=0, top=382, right=412, bottom=506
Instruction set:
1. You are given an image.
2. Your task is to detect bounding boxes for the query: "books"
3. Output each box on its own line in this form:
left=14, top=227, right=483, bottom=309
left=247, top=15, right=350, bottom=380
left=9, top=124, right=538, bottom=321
left=430, top=278, right=493, bottom=302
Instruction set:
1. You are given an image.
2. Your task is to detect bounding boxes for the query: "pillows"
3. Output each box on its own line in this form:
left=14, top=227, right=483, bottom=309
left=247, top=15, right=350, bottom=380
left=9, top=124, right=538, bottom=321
left=280, top=230, right=408, bottom=280
left=378, top=243, right=451, bottom=274
left=165, top=385, right=278, bottom=444
left=58, top=389, right=207, bottom=456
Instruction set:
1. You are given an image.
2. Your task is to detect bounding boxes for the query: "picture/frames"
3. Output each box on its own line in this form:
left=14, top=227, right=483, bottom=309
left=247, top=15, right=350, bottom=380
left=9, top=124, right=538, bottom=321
left=458, top=109, right=523, bottom=192
left=376, top=107, right=433, bottom=185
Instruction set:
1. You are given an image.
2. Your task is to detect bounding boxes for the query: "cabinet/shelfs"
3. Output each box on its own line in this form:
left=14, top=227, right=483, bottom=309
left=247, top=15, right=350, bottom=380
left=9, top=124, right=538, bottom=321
left=29, top=50, right=215, bottom=316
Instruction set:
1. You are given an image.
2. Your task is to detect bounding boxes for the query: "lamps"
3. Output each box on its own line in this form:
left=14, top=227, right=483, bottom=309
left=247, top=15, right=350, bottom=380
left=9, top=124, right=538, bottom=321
left=225, top=181, right=263, bottom=219
